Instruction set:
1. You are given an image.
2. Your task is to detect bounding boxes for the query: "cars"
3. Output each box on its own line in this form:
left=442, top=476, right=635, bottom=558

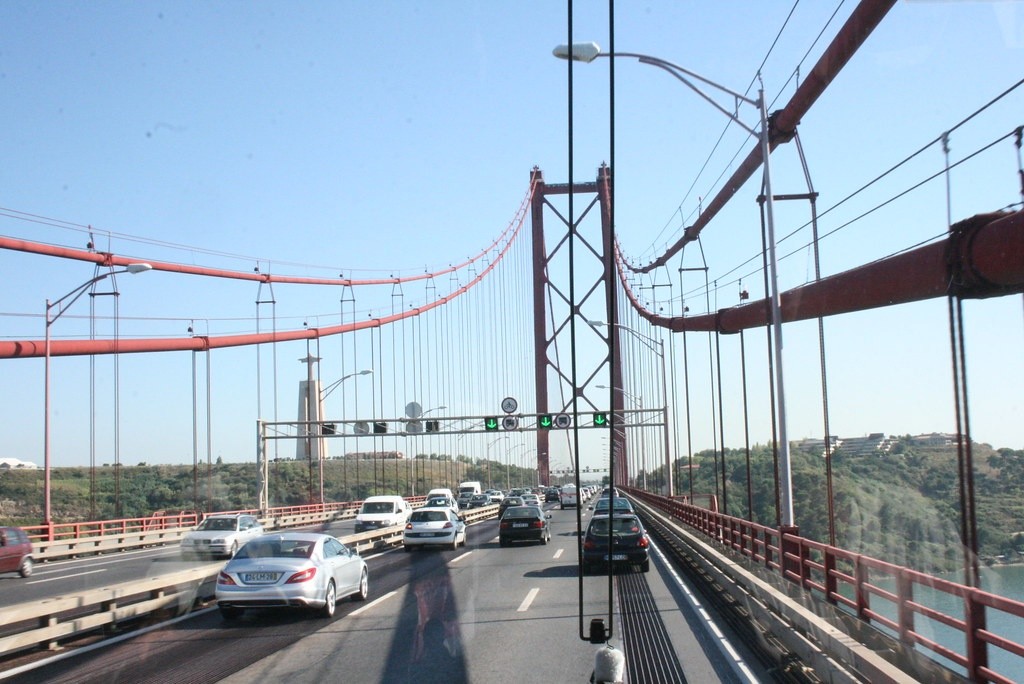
left=402, top=507, right=468, bottom=551
left=426, top=498, right=458, bottom=514
left=581, top=514, right=650, bottom=571
left=498, top=506, right=553, bottom=546
left=591, top=497, right=638, bottom=519
left=426, top=489, right=454, bottom=503
left=0, top=528, right=37, bottom=578
left=214, top=534, right=370, bottom=620
left=179, top=512, right=264, bottom=562
left=498, top=497, right=525, bottom=521
left=356, top=495, right=412, bottom=533
left=600, top=487, right=621, bottom=499
left=581, top=484, right=602, bottom=503
left=459, top=482, right=561, bottom=513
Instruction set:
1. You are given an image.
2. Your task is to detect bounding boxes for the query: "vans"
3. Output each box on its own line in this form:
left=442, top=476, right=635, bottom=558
left=559, top=485, right=583, bottom=510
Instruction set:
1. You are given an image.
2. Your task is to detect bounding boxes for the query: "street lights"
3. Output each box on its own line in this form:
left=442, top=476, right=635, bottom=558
left=596, top=384, right=648, bottom=491
left=506, top=442, right=528, bottom=492
left=486, top=436, right=510, bottom=491
left=319, top=369, right=374, bottom=504
left=552, top=40, right=794, bottom=548
left=588, top=319, right=679, bottom=500
left=409, top=406, right=448, bottom=497
left=40, top=260, right=154, bottom=539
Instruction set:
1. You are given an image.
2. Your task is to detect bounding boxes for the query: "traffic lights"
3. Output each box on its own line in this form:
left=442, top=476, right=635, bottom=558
left=592, top=412, right=606, bottom=427
left=539, top=415, right=553, bottom=429
left=484, top=417, right=500, bottom=432
